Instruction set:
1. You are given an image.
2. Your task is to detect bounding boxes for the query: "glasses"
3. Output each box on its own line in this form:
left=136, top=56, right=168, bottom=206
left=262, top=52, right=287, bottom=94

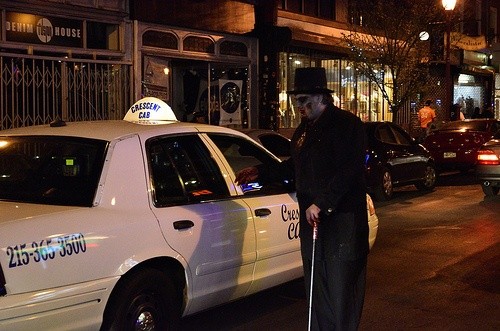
left=295, top=95, right=316, bottom=104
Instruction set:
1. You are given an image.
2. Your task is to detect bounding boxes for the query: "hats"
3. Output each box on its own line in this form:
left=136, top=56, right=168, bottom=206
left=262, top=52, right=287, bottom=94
left=286, top=67, right=334, bottom=94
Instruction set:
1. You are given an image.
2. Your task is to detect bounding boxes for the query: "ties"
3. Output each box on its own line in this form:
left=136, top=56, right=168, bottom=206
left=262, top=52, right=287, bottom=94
left=296, top=122, right=311, bottom=150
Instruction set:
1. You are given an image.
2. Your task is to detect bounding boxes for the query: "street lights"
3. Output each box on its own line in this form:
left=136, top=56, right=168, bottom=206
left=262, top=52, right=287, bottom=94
left=440, top=0, right=458, bottom=123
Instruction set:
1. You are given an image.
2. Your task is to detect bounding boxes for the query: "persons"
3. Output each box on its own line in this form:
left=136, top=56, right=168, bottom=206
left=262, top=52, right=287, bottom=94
left=416, top=99, right=436, bottom=131
left=234, top=68, right=368, bottom=331
left=450, top=103, right=493, bottom=121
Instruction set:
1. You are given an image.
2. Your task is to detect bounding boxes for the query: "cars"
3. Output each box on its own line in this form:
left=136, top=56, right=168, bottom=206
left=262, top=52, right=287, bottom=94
left=422, top=118, right=500, bottom=176
left=218, top=129, right=292, bottom=173
left=475, top=129, right=500, bottom=197
left=0, top=96, right=379, bottom=331
left=362, top=121, right=437, bottom=201
